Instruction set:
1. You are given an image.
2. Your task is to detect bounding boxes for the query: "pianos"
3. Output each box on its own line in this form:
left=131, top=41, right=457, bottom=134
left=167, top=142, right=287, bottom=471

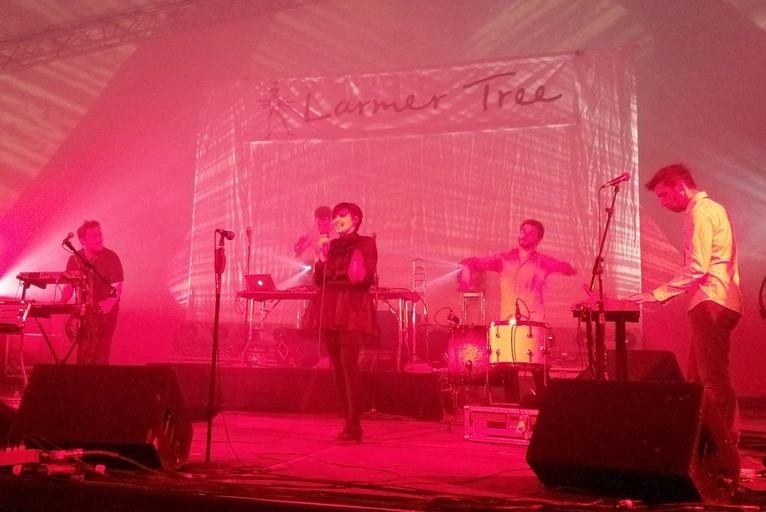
left=18, top=305, right=103, bottom=318
left=16, top=272, right=93, bottom=289
left=573, top=297, right=640, bottom=321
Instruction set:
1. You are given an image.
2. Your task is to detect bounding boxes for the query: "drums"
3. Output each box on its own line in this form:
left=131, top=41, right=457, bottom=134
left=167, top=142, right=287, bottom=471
left=490, top=319, right=553, bottom=371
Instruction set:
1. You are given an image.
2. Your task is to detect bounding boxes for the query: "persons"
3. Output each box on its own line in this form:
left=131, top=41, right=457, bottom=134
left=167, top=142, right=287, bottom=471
left=291, top=205, right=336, bottom=285
left=51, top=218, right=125, bottom=366
left=625, top=164, right=744, bottom=494
left=460, top=218, right=591, bottom=409
left=298, top=202, right=380, bottom=443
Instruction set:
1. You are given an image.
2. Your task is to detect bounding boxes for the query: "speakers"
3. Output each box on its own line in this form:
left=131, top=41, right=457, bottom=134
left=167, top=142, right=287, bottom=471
left=16, top=364, right=193, bottom=472
left=526, top=378, right=739, bottom=504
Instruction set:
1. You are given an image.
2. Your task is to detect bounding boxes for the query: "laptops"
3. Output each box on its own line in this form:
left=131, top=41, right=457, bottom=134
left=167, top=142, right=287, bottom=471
left=243, top=274, right=289, bottom=291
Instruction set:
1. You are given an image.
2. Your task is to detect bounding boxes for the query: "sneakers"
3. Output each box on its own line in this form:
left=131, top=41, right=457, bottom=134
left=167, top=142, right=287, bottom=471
left=338, top=427, right=362, bottom=443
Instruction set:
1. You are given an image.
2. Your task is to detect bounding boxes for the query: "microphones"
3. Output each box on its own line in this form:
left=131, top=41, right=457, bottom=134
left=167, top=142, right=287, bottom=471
left=603, top=172, right=630, bottom=188
left=246, top=227, right=253, bottom=242
left=218, top=229, right=235, bottom=240
left=319, top=233, right=329, bottom=263
left=515, top=303, right=521, bottom=320
left=63, top=232, right=74, bottom=243
